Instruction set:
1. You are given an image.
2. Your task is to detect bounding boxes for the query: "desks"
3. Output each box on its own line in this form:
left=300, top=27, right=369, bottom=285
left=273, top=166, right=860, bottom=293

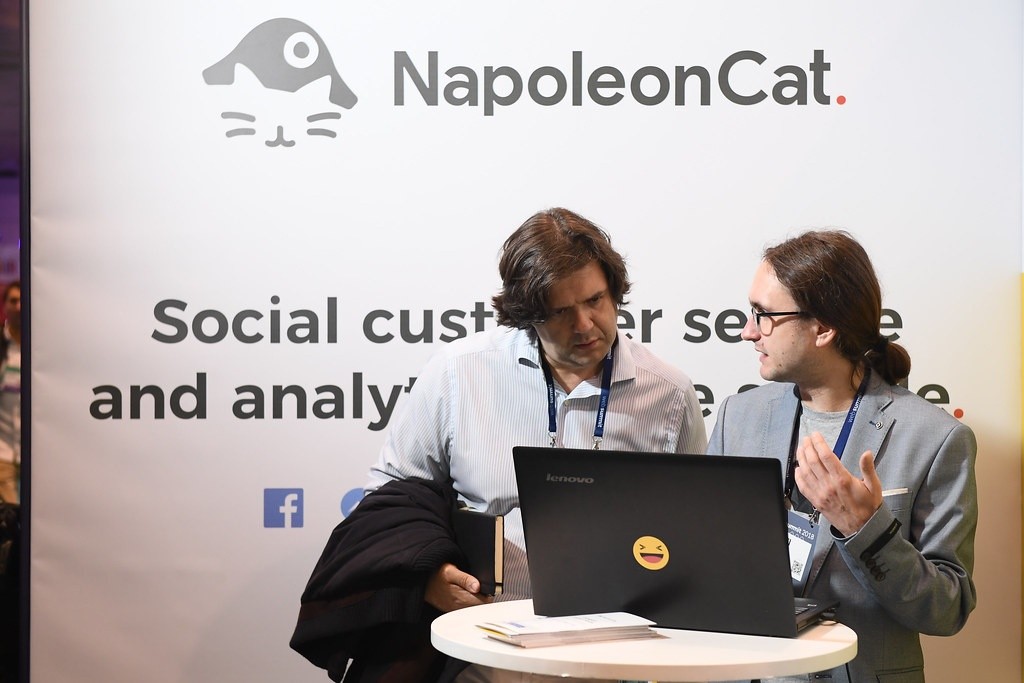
left=430, top=598, right=858, bottom=683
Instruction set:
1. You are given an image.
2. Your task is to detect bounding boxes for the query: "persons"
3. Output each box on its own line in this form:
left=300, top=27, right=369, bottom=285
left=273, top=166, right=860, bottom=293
left=358, top=208, right=709, bottom=683
left=704, top=228, right=977, bottom=683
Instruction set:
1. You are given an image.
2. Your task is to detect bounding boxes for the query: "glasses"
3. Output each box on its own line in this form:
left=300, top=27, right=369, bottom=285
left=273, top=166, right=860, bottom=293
left=751, top=307, right=807, bottom=328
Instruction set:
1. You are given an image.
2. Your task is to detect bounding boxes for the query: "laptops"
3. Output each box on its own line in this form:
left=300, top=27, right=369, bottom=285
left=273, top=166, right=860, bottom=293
left=513, top=447, right=840, bottom=638
